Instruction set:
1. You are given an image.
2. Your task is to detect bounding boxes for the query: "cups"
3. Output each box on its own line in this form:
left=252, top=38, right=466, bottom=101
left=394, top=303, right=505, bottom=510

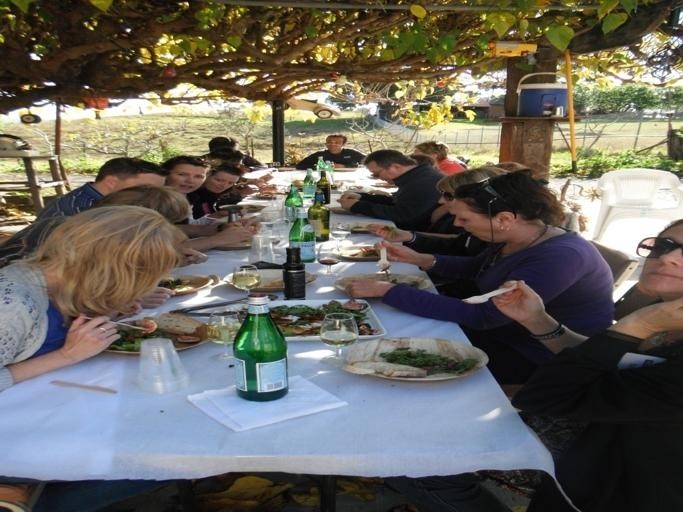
left=249, top=234, right=275, bottom=264
left=136, top=338, right=191, bottom=395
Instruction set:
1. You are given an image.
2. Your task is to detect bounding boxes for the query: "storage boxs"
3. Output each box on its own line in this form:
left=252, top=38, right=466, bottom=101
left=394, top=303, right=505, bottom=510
left=517, top=72, right=568, bottom=118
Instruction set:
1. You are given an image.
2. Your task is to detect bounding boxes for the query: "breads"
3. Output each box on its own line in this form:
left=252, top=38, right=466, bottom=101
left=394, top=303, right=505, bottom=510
left=158, top=312, right=201, bottom=334
left=342, top=361, right=376, bottom=375
left=373, top=362, right=428, bottom=379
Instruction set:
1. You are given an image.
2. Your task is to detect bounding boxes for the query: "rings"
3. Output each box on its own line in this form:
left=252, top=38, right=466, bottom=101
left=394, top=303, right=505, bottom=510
left=97, top=327, right=107, bottom=332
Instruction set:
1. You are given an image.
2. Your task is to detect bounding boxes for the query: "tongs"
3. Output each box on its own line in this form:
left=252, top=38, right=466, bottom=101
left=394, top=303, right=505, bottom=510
left=169, top=294, right=279, bottom=317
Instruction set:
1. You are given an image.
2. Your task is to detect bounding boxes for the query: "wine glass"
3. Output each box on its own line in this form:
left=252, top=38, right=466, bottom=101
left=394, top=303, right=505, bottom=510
left=331, top=221, right=352, bottom=257
left=317, top=243, right=343, bottom=279
left=207, top=310, right=244, bottom=370
left=232, top=263, right=262, bottom=296
left=273, top=161, right=281, bottom=172
left=283, top=205, right=297, bottom=226
left=330, top=161, right=335, bottom=169
left=319, top=312, right=359, bottom=365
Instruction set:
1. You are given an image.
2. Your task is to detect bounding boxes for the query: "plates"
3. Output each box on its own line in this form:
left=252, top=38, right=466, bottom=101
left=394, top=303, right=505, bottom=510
left=344, top=337, right=490, bottom=383
left=336, top=226, right=373, bottom=234
left=333, top=241, right=380, bottom=262
left=203, top=212, right=264, bottom=223
left=105, top=341, right=210, bottom=356
left=223, top=268, right=317, bottom=292
left=336, top=275, right=433, bottom=293
left=334, top=168, right=356, bottom=172
left=218, top=203, right=266, bottom=213
left=158, top=274, right=214, bottom=295
left=330, top=207, right=352, bottom=215
left=266, top=299, right=386, bottom=342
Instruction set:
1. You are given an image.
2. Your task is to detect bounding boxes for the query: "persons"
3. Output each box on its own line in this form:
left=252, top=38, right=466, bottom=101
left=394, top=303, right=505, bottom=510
left=345, top=173, right=615, bottom=402
left=0, top=205, right=177, bottom=512
left=296, top=136, right=366, bottom=170
left=338, top=140, right=531, bottom=279
left=193, top=221, right=683, bottom=512
left=1, top=135, right=263, bottom=309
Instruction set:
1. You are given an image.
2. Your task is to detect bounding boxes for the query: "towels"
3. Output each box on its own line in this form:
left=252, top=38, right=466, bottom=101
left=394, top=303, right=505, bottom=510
left=187, top=375, right=347, bottom=433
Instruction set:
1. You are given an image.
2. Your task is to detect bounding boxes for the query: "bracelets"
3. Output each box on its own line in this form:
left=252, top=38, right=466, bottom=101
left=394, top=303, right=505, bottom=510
left=422, top=255, right=437, bottom=271
left=532, top=323, right=565, bottom=340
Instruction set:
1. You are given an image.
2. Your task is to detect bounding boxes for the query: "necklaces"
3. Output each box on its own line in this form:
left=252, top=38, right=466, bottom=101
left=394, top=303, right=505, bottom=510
left=504, top=224, right=549, bottom=258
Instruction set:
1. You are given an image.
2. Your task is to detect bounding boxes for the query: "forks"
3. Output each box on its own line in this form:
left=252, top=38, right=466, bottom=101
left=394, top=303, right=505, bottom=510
left=459, top=285, right=516, bottom=307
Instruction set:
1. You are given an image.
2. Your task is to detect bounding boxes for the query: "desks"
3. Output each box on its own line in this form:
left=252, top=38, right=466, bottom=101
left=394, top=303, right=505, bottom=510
left=0, top=147, right=67, bottom=211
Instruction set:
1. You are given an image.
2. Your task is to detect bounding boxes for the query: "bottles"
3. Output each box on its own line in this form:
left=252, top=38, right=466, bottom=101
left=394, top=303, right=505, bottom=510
left=307, top=189, right=330, bottom=242
left=317, top=155, right=326, bottom=172
left=283, top=184, right=303, bottom=224
left=233, top=293, right=288, bottom=402
left=288, top=207, right=316, bottom=263
left=303, top=168, right=317, bottom=200
left=325, top=160, right=333, bottom=173
left=282, top=247, right=306, bottom=301
left=228, top=210, right=239, bottom=222
left=316, top=171, right=332, bottom=204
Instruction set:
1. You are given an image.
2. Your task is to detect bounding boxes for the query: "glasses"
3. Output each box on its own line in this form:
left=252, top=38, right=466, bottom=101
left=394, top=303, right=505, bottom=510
left=370, top=169, right=383, bottom=178
left=636, top=237, right=682, bottom=259
left=477, top=178, right=505, bottom=202
left=436, top=190, right=456, bottom=202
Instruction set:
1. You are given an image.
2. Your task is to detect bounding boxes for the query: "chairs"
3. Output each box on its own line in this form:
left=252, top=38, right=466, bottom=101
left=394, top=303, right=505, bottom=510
left=592, top=169, right=683, bottom=243
left=615, top=282, right=662, bottom=322
left=590, top=238, right=640, bottom=295
